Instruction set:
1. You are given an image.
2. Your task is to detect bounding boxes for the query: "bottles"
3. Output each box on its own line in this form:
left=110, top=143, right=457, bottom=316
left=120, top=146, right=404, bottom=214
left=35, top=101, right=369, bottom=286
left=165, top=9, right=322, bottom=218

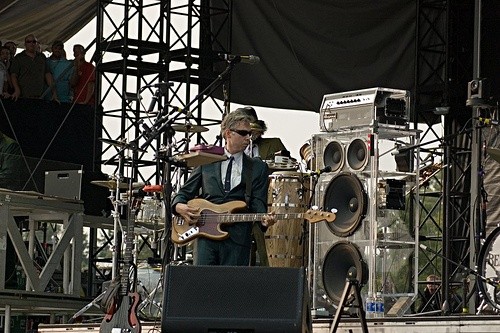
left=375, top=292, right=384, bottom=317
left=366, top=292, right=375, bottom=318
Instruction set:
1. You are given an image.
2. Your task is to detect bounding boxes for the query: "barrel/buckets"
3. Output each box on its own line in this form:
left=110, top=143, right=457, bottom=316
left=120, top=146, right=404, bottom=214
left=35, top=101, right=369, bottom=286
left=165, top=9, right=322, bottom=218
left=266, top=171, right=311, bottom=268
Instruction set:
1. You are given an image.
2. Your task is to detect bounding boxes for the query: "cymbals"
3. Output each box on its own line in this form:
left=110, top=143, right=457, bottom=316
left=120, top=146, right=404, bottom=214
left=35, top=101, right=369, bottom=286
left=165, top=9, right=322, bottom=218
left=91, top=180, right=142, bottom=190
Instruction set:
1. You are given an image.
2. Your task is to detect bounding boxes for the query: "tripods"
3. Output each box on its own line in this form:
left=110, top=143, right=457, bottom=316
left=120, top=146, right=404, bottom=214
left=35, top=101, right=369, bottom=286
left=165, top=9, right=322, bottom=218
left=66, top=148, right=162, bottom=324
left=389, top=121, right=494, bottom=318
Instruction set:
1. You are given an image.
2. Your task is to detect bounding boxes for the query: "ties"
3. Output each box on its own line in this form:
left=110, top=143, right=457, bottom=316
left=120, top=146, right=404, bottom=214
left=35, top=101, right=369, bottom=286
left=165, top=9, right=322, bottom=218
left=225, top=156, right=234, bottom=194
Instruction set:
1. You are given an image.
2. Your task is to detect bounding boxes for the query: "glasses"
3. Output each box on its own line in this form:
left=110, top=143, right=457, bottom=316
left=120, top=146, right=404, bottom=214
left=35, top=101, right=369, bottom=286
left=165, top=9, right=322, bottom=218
left=230, top=129, right=252, bottom=136
left=25, top=40, right=37, bottom=43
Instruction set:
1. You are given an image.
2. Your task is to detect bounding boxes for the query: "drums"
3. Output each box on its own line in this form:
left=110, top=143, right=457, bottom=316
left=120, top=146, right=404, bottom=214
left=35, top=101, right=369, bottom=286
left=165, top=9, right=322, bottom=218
left=134, top=197, right=165, bottom=230
left=128, top=268, right=164, bottom=320
left=476, top=226, right=500, bottom=309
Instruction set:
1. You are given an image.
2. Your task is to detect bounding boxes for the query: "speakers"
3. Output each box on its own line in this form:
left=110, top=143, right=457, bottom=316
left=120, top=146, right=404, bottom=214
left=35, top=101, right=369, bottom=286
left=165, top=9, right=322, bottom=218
left=46, top=169, right=127, bottom=219
left=161, top=265, right=313, bottom=333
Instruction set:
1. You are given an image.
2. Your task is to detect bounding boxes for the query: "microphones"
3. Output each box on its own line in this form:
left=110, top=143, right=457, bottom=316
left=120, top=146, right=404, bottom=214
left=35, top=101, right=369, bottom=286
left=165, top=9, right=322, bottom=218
left=167, top=104, right=192, bottom=116
left=219, top=53, right=260, bottom=64
left=142, top=83, right=174, bottom=88
left=303, top=166, right=332, bottom=178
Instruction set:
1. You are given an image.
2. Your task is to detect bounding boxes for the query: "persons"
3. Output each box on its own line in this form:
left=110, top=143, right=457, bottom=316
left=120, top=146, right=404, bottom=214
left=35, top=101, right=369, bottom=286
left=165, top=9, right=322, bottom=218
left=381, top=275, right=462, bottom=314
left=0, top=35, right=96, bottom=107
left=245, top=109, right=286, bottom=160
left=170, top=110, right=277, bottom=267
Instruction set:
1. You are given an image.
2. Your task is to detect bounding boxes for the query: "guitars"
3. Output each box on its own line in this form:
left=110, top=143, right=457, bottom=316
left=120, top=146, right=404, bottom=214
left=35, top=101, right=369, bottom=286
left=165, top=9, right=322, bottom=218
left=99, top=208, right=142, bottom=333
left=170, top=197, right=336, bottom=245
